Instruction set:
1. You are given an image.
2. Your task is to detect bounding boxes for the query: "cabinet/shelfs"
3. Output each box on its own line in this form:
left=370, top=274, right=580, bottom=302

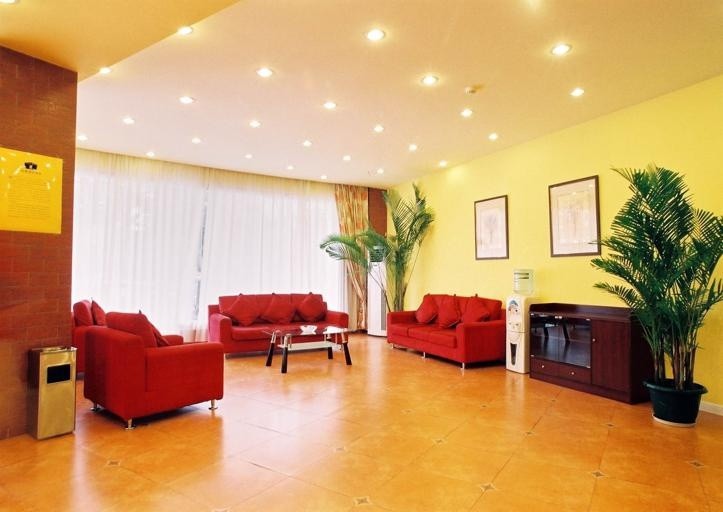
left=526, top=302, right=669, bottom=405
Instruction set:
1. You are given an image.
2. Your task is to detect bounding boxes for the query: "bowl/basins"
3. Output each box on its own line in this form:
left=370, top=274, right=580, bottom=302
left=299, top=331, right=316, bottom=334
left=298, top=324, right=317, bottom=332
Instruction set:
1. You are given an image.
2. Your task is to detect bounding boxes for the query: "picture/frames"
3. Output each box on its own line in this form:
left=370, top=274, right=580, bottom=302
left=473, top=193, right=510, bottom=261
left=548, top=174, right=603, bottom=258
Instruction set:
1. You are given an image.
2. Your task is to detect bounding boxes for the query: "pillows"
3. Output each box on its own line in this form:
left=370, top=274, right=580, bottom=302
left=222, top=292, right=265, bottom=326
left=258, top=294, right=297, bottom=326
left=457, top=293, right=489, bottom=323
left=294, top=293, right=326, bottom=324
left=435, top=293, right=462, bottom=329
left=89, top=299, right=107, bottom=325
left=413, top=293, right=438, bottom=325
left=136, top=309, right=171, bottom=347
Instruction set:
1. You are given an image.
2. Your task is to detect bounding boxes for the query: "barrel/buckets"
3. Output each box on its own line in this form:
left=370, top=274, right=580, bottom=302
left=513, top=269, right=533, bottom=296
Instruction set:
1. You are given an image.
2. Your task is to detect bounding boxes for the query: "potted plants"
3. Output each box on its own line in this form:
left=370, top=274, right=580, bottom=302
left=587, top=160, right=723, bottom=429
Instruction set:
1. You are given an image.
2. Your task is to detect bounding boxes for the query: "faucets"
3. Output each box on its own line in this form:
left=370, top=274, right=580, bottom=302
left=506, top=306, right=511, bottom=314
left=514, top=306, right=519, bottom=314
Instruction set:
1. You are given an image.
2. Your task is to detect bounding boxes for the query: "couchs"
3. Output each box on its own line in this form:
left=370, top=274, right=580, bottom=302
left=385, top=293, right=506, bottom=369
left=70, top=297, right=92, bottom=374
left=206, top=294, right=349, bottom=355
left=84, top=309, right=224, bottom=430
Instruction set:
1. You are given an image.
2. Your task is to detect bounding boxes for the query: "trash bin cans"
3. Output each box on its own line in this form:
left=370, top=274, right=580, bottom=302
left=26, top=344, right=78, bottom=441
